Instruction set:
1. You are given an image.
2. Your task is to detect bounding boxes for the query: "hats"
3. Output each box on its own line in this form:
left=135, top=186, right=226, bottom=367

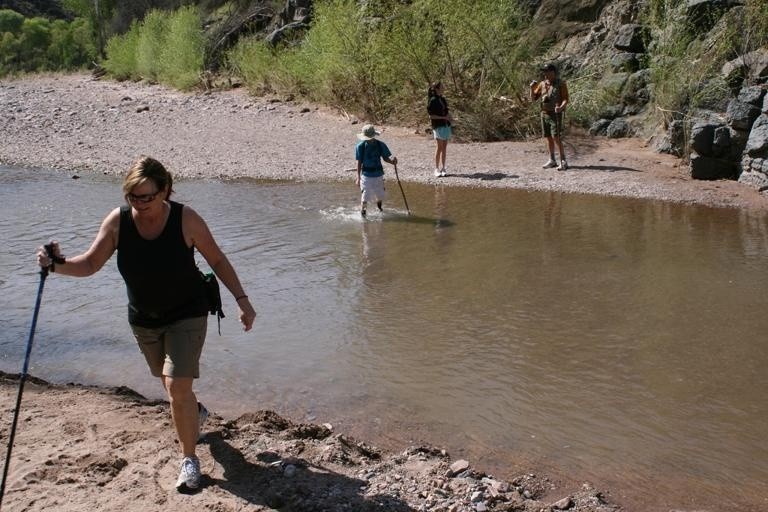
left=356, top=123, right=381, bottom=142
left=540, top=63, right=557, bottom=71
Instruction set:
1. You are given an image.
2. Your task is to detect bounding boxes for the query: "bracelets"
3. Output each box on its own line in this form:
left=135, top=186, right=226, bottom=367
left=235, top=296, right=248, bottom=302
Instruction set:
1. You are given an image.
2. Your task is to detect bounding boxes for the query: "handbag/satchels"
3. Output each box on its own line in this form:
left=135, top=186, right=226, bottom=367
left=204, top=272, right=226, bottom=319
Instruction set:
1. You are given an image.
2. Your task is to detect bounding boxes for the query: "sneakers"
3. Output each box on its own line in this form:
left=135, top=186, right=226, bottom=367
left=434, top=168, right=441, bottom=177
left=556, top=159, right=568, bottom=171
left=542, top=159, right=557, bottom=169
left=197, top=401, right=208, bottom=443
left=440, top=168, right=447, bottom=177
left=175, top=454, right=201, bottom=492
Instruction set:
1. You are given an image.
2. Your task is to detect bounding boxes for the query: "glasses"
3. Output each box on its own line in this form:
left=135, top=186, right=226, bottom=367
left=127, top=190, right=161, bottom=204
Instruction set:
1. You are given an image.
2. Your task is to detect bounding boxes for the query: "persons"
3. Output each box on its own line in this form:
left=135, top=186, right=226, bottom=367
left=360, top=221, right=396, bottom=279
left=425, top=81, right=454, bottom=177
left=355, top=125, right=397, bottom=216
left=529, top=63, right=570, bottom=170
left=540, top=190, right=565, bottom=271
left=432, top=185, right=454, bottom=272
left=40, top=158, right=255, bottom=494
